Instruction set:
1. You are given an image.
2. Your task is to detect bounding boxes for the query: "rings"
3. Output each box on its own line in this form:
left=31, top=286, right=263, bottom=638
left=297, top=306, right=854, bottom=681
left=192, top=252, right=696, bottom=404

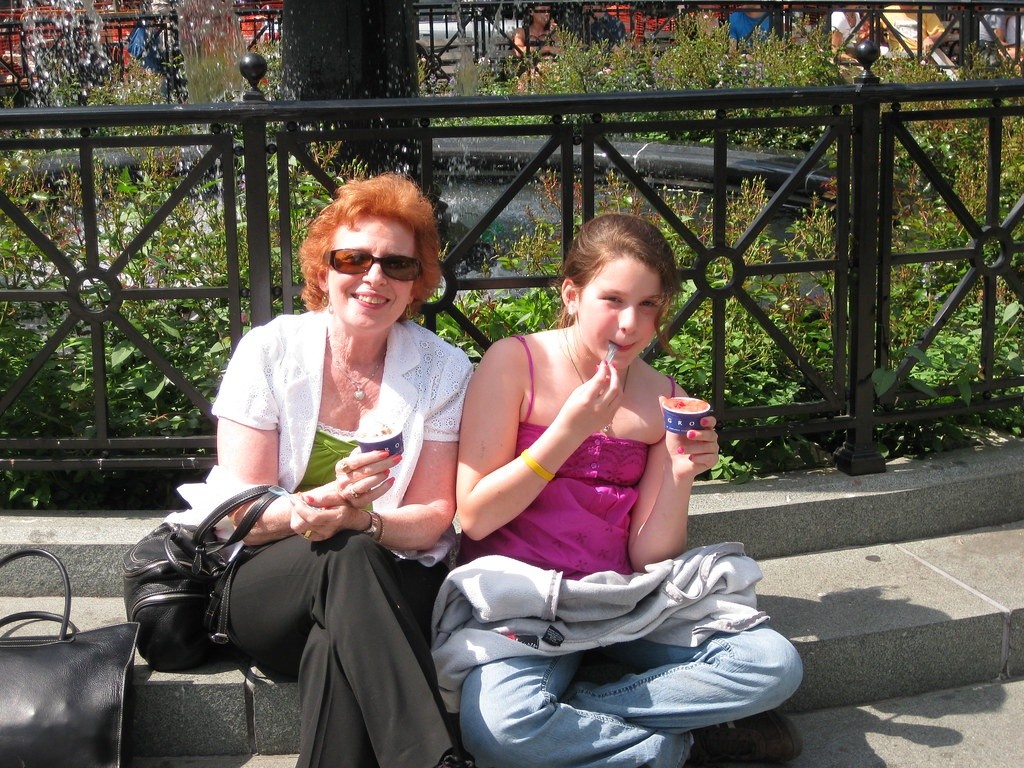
left=302, top=529, right=311, bottom=539
left=339, top=461, right=352, bottom=474
left=350, top=486, right=361, bottom=497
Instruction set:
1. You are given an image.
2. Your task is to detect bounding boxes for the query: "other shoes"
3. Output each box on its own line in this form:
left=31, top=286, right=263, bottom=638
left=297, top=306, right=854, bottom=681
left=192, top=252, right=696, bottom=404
left=690, top=711, right=802, bottom=768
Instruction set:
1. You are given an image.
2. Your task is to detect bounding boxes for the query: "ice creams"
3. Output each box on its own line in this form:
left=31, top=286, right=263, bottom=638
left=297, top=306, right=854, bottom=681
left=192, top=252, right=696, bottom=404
left=354, top=423, right=402, bottom=443
left=663, top=397, right=710, bottom=414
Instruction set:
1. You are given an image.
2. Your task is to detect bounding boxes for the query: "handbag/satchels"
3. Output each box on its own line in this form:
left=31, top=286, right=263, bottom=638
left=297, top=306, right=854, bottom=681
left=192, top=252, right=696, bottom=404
left=0, top=549, right=140, bottom=768
left=123, top=484, right=289, bottom=673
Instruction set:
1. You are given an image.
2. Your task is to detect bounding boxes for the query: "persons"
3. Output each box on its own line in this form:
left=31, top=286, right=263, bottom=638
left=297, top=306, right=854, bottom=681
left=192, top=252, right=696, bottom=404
left=831, top=4, right=949, bottom=65
left=506, top=2, right=564, bottom=89
left=211, top=172, right=474, bottom=768
left=454, top=213, right=804, bottom=768
left=728, top=3, right=775, bottom=54
left=979, top=7, right=1024, bottom=67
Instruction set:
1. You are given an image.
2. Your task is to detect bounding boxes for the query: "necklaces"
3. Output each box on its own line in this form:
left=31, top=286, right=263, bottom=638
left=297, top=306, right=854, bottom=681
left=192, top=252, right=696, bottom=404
left=325, top=351, right=385, bottom=401
left=564, top=328, right=630, bottom=432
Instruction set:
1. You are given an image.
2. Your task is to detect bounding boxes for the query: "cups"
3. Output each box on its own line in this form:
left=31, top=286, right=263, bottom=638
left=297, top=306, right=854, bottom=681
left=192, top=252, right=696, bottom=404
left=354, top=418, right=405, bottom=461
left=661, top=397, right=710, bottom=435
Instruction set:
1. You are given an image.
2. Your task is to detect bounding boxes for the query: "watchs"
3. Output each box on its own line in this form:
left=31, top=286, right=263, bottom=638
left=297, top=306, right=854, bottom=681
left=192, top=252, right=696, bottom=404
left=362, top=509, right=378, bottom=537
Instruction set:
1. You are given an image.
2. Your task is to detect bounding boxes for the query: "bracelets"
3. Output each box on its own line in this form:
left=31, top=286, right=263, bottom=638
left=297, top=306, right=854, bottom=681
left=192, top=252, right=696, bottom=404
left=371, top=511, right=385, bottom=543
left=521, top=449, right=555, bottom=481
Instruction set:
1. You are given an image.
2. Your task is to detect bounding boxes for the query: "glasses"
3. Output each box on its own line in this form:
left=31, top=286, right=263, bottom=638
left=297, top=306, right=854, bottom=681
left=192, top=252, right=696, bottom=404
left=326, top=248, right=422, bottom=281
